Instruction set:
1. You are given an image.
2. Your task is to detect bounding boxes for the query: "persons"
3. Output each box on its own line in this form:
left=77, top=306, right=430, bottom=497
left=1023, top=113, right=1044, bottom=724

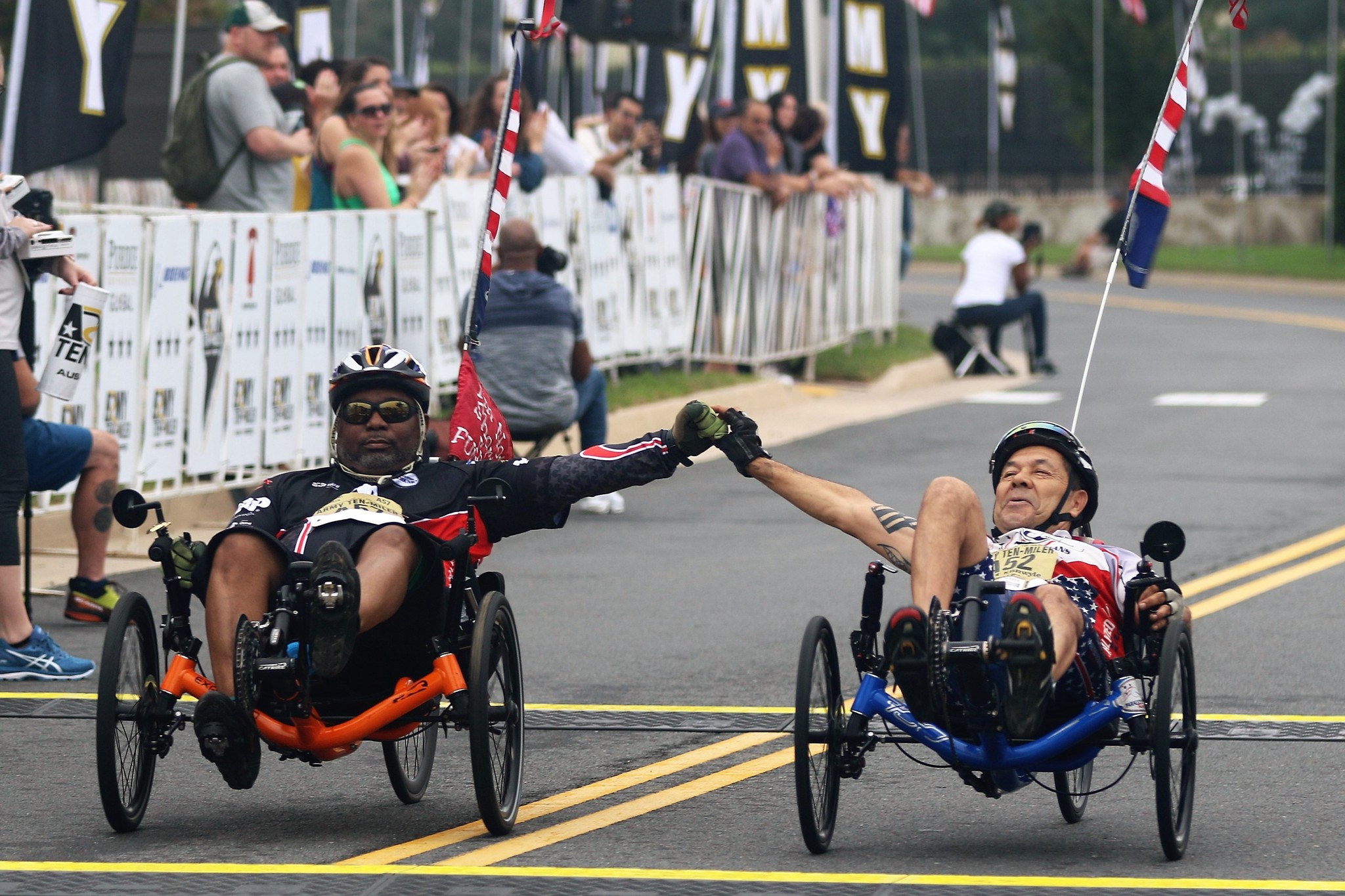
left=13, top=338, right=140, bottom=626
left=459, top=219, right=625, bottom=514
left=170, top=345, right=728, bottom=789
left=954, top=200, right=1061, bottom=379
left=711, top=403, right=1188, bottom=747
left=205, top=1, right=932, bottom=384
left=0, top=171, right=98, bottom=681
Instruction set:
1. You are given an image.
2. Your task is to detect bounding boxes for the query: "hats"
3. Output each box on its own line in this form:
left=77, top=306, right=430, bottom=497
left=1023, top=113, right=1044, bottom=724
left=223, top=0, right=291, bottom=36
left=392, top=72, right=419, bottom=98
left=711, top=96, right=739, bottom=123
left=983, top=200, right=1022, bottom=221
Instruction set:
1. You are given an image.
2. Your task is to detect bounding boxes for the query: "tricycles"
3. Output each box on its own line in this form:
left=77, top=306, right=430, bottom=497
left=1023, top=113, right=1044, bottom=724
left=95, top=488, right=526, bottom=837
left=793, top=523, right=1198, bottom=862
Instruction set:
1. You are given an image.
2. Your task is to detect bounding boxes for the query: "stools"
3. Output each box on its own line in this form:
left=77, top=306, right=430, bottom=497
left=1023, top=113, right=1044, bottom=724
left=949, top=319, right=1014, bottom=376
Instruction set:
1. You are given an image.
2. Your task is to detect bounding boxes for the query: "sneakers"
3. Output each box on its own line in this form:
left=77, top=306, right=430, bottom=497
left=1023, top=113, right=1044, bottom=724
left=303, top=539, right=361, bottom=677
left=0, top=624, right=95, bottom=682
left=999, top=592, right=1054, bottom=737
left=64, top=578, right=135, bottom=625
left=883, top=604, right=937, bottom=723
left=193, top=688, right=261, bottom=790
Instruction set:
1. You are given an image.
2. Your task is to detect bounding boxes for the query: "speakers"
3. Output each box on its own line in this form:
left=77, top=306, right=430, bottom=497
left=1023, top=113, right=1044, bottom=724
left=560, top=0, right=693, bottom=49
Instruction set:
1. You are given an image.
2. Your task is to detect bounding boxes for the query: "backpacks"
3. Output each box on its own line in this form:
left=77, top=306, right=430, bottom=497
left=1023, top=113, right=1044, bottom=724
left=159, top=58, right=262, bottom=202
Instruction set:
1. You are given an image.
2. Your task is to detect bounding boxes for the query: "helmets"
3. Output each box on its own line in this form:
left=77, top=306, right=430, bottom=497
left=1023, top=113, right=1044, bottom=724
left=989, top=420, right=1099, bottom=530
left=328, top=343, right=430, bottom=417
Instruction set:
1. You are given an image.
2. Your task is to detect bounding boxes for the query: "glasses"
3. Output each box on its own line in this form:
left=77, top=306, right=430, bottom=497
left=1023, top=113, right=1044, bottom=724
left=354, top=103, right=391, bottom=118
left=613, top=109, right=642, bottom=123
left=338, top=400, right=418, bottom=424
left=357, top=79, right=391, bottom=92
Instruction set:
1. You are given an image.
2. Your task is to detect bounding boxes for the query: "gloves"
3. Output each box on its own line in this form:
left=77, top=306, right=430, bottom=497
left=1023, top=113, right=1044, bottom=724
left=160, top=537, right=207, bottom=591
left=671, top=399, right=729, bottom=458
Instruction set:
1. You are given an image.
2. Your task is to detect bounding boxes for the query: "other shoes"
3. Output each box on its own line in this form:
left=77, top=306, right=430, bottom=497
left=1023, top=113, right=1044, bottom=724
left=1030, top=362, right=1056, bottom=381
